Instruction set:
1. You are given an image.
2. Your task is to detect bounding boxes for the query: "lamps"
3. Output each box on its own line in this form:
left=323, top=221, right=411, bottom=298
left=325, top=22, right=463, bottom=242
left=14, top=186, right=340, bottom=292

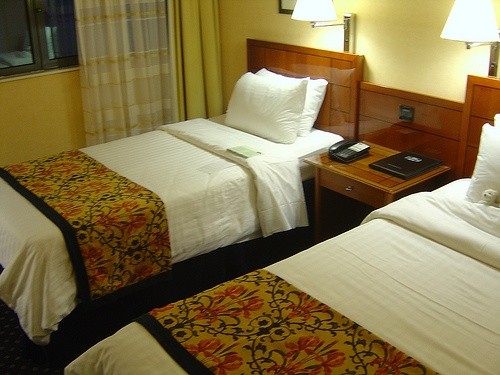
left=440, top=1, right=500, bottom=75
left=292, top=0, right=354, bottom=52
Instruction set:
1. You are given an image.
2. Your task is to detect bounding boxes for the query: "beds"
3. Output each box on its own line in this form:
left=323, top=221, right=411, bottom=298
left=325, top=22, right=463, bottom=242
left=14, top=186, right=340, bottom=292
left=63, top=75, right=500, bottom=375
left=0, top=36, right=364, bottom=345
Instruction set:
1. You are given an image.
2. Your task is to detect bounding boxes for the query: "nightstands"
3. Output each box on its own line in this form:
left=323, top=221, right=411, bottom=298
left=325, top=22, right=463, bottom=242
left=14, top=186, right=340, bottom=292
left=304, top=140, right=451, bottom=241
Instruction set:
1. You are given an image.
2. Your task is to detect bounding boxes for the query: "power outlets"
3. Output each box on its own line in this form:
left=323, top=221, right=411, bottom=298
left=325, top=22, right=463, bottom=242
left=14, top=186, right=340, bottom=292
left=399, top=104, right=414, bottom=121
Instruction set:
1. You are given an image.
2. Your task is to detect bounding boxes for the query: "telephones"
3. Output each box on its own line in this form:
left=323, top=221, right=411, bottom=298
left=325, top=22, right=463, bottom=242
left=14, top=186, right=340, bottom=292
left=326, top=140, right=372, bottom=165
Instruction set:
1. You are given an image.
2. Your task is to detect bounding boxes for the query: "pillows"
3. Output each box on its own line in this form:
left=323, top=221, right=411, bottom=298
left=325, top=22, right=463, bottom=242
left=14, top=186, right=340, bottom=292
left=224, top=68, right=329, bottom=145
left=467, top=114, right=500, bottom=206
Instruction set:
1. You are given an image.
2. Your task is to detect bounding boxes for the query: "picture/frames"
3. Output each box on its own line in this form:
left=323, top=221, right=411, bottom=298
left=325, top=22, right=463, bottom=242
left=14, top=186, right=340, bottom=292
left=278, top=0, right=297, bottom=14
